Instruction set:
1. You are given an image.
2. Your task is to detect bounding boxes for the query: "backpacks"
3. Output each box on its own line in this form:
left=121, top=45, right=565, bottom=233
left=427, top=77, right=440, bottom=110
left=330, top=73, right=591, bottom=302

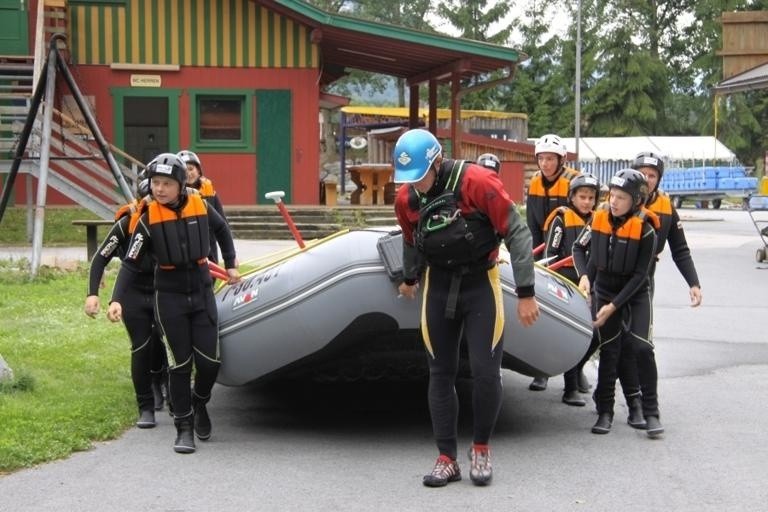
left=409, top=158, right=478, bottom=274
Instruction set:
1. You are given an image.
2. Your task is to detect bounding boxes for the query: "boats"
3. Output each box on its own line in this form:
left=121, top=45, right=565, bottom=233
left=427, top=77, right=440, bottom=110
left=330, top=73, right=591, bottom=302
left=212, top=219, right=597, bottom=396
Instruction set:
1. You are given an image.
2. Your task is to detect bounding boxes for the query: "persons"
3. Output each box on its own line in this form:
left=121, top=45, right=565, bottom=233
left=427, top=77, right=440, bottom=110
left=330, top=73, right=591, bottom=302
left=476, top=153, right=500, bottom=176
left=148, top=321, right=170, bottom=411
left=106, top=152, right=241, bottom=455
left=541, top=171, right=599, bottom=407
left=526, top=133, right=579, bottom=389
left=390, top=129, right=539, bottom=486
left=176, top=149, right=237, bottom=292
left=85, top=179, right=195, bottom=428
left=591, top=150, right=703, bottom=430
left=570, top=167, right=666, bottom=437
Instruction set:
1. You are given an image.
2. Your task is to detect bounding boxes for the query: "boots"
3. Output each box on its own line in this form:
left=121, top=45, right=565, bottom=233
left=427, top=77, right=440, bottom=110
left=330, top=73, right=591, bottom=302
left=129, top=335, right=169, bottom=429
left=190, top=341, right=220, bottom=441
left=628, top=330, right=665, bottom=437
left=167, top=352, right=195, bottom=455
left=589, top=330, right=624, bottom=436
left=625, top=388, right=649, bottom=431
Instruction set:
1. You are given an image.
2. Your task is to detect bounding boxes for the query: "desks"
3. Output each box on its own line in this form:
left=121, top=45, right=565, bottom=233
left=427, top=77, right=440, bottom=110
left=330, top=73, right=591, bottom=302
left=345, top=164, right=395, bottom=209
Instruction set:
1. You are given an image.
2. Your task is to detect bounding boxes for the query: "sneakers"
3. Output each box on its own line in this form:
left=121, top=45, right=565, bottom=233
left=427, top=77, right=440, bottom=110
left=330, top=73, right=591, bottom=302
left=465, top=438, right=494, bottom=488
left=528, top=374, right=548, bottom=392
left=421, top=454, right=463, bottom=488
left=560, top=369, right=593, bottom=408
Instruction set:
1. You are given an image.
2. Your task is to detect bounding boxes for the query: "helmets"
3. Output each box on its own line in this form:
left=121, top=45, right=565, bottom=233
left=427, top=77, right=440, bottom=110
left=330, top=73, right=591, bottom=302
left=532, top=133, right=567, bottom=160
left=607, top=167, right=649, bottom=213
left=629, top=151, right=664, bottom=180
left=143, top=151, right=189, bottom=198
left=391, top=127, right=443, bottom=185
left=175, top=150, right=204, bottom=178
left=475, top=152, right=500, bottom=175
left=134, top=168, right=150, bottom=200
left=565, top=172, right=600, bottom=211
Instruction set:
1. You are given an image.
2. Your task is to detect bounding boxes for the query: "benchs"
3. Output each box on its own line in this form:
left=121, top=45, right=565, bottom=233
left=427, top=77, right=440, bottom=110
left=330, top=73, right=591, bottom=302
left=320, top=180, right=338, bottom=206
left=72, top=219, right=120, bottom=263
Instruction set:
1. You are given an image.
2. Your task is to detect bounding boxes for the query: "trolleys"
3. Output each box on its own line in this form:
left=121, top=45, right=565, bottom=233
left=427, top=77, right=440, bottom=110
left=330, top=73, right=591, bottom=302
left=741, top=193, right=767, bottom=263
